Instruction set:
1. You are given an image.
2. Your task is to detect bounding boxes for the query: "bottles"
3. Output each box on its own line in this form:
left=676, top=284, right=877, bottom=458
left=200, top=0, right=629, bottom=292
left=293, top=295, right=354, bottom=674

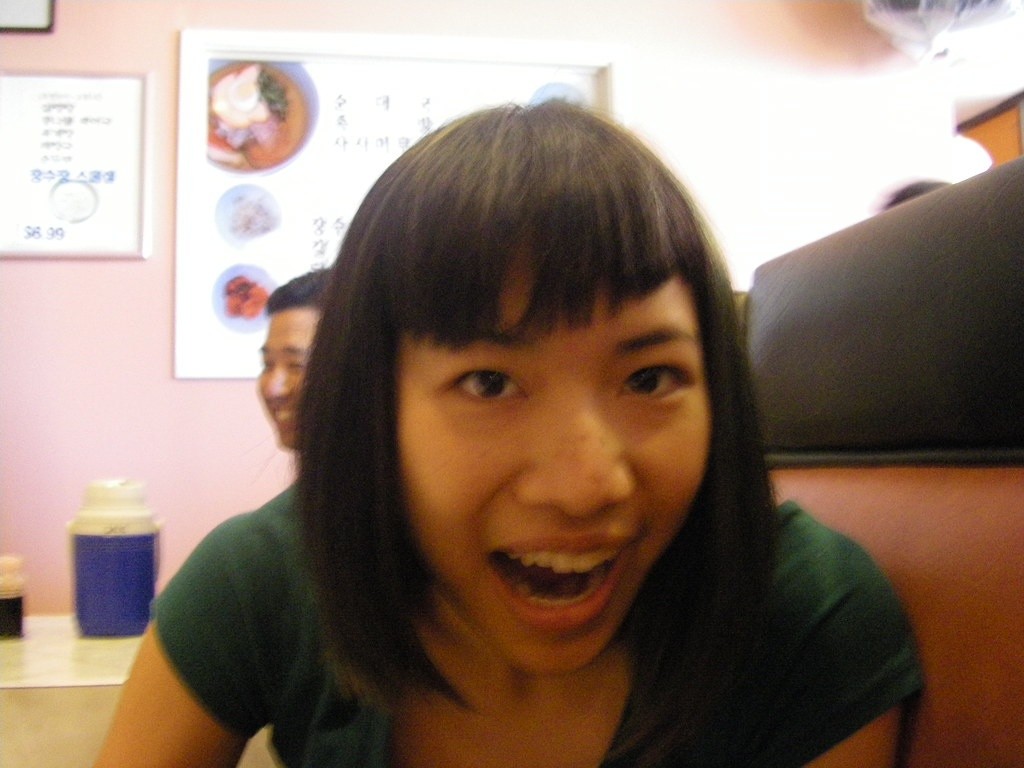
left=0, top=556, right=25, bottom=639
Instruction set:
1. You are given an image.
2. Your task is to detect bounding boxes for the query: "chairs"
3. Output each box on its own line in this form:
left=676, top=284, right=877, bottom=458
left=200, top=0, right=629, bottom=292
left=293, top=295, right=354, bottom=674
left=745, top=154, right=1024, bottom=768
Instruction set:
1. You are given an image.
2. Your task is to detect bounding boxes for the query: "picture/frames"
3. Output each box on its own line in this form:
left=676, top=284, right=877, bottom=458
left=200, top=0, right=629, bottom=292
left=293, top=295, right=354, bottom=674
left=0, top=0, right=56, bottom=31
left=174, top=29, right=639, bottom=380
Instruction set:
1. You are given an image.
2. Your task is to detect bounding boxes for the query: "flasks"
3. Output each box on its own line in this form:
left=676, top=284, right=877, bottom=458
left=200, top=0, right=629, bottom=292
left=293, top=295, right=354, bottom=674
left=67, top=478, right=160, bottom=638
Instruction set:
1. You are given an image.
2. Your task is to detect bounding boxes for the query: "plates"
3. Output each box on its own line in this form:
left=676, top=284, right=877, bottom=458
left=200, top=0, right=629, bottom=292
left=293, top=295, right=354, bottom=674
left=215, top=183, right=282, bottom=249
left=211, top=264, right=278, bottom=333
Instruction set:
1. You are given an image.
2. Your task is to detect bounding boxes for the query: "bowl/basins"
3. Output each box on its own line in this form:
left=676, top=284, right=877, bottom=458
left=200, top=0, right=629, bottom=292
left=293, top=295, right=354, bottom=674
left=207, top=59, right=320, bottom=180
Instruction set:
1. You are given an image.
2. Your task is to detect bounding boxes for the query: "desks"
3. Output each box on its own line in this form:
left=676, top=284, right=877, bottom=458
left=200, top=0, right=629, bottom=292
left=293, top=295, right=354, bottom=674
left=1, top=614, right=287, bottom=768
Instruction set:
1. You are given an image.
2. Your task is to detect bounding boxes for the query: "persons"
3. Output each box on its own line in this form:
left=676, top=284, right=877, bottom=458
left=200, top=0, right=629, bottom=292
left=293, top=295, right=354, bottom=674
left=258, top=264, right=335, bottom=452
left=96, top=102, right=919, bottom=768
